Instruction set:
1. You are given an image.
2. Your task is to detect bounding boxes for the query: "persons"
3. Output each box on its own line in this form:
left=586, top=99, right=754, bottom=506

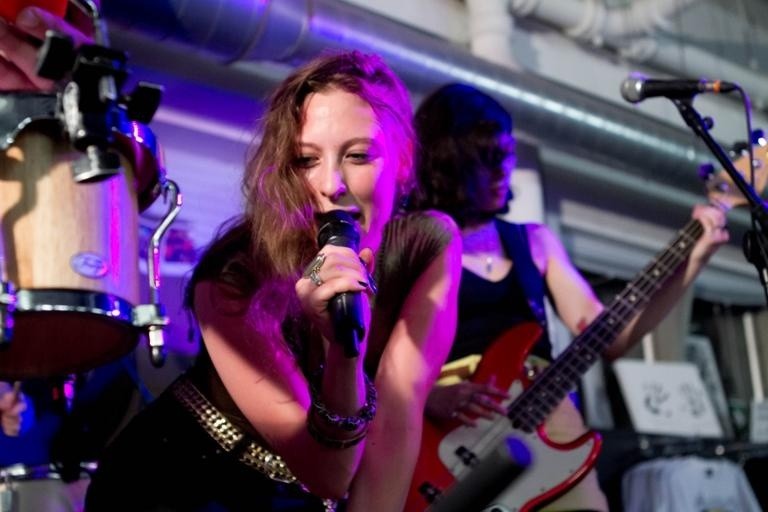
left=401, top=84, right=730, bottom=511
left=0, top=380, right=26, bottom=437
left=84, top=51, right=463, bottom=512
left=0, top=1, right=95, bottom=94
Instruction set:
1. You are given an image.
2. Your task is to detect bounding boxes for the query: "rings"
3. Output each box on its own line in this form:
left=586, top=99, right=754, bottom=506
left=452, top=411, right=458, bottom=418
left=303, top=254, right=324, bottom=286
left=460, top=399, right=468, bottom=407
left=721, top=224, right=728, bottom=231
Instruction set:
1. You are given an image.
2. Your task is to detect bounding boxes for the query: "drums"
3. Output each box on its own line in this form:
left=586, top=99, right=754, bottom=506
left=1, top=89, right=182, bottom=382
left=0, top=461, right=97, bottom=512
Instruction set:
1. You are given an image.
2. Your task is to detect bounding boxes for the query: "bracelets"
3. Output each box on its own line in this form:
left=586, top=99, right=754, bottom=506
left=306, top=407, right=370, bottom=450
left=308, top=365, right=378, bottom=431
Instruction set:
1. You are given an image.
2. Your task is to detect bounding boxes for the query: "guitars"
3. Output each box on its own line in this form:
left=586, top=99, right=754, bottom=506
left=401, top=130, right=768, bottom=512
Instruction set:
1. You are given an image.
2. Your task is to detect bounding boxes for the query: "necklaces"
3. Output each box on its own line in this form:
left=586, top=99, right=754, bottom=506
left=464, top=235, right=495, bottom=273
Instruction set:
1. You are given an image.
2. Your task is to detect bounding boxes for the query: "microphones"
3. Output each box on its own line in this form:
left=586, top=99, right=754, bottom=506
left=618, top=73, right=724, bottom=104
left=316, top=211, right=367, bottom=359
